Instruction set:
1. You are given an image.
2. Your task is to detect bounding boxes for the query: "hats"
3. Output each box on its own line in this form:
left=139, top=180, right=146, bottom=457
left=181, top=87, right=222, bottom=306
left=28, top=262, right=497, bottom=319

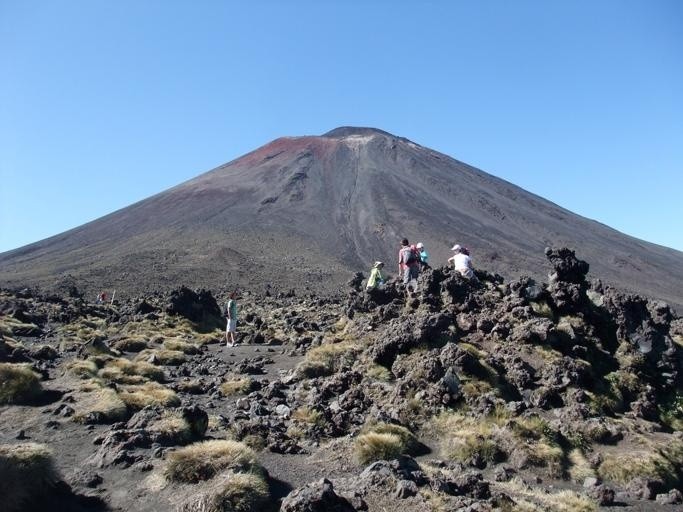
left=450, top=244, right=461, bottom=251
left=373, top=261, right=384, bottom=268
left=416, top=243, right=423, bottom=249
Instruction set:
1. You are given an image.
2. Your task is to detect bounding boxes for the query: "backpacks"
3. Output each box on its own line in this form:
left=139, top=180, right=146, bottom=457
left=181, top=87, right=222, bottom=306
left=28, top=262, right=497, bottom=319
left=402, top=247, right=416, bottom=265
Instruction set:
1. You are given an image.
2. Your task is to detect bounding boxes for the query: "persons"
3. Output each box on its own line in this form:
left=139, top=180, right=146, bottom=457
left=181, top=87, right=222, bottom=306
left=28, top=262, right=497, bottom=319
left=95, top=294, right=100, bottom=304
left=224, top=291, right=238, bottom=348
left=99, top=291, right=104, bottom=302
left=451, top=245, right=461, bottom=255
left=415, top=242, right=428, bottom=262
left=397, top=238, right=422, bottom=283
left=366, top=260, right=385, bottom=293
left=447, top=246, right=481, bottom=281
